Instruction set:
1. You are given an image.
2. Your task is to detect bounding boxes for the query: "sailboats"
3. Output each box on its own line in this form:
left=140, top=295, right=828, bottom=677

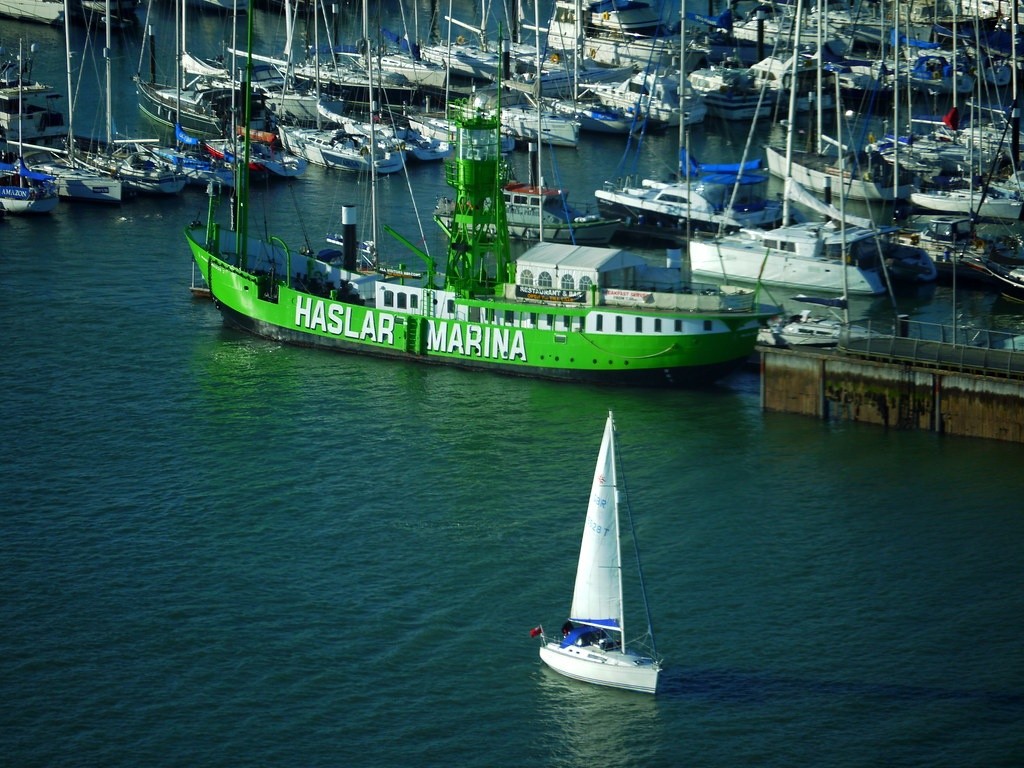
left=0, top=1, right=1022, bottom=385
left=528, top=409, right=670, bottom=694
